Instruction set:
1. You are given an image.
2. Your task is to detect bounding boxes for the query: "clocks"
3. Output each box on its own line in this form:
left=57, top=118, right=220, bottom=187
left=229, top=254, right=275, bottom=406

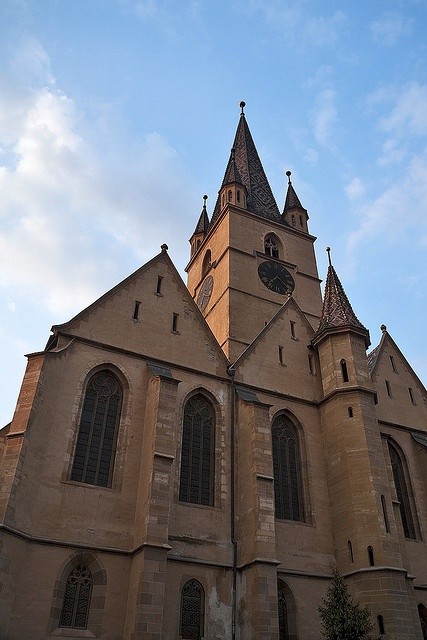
left=193, top=261, right=218, bottom=315
left=253, top=250, right=300, bottom=305
